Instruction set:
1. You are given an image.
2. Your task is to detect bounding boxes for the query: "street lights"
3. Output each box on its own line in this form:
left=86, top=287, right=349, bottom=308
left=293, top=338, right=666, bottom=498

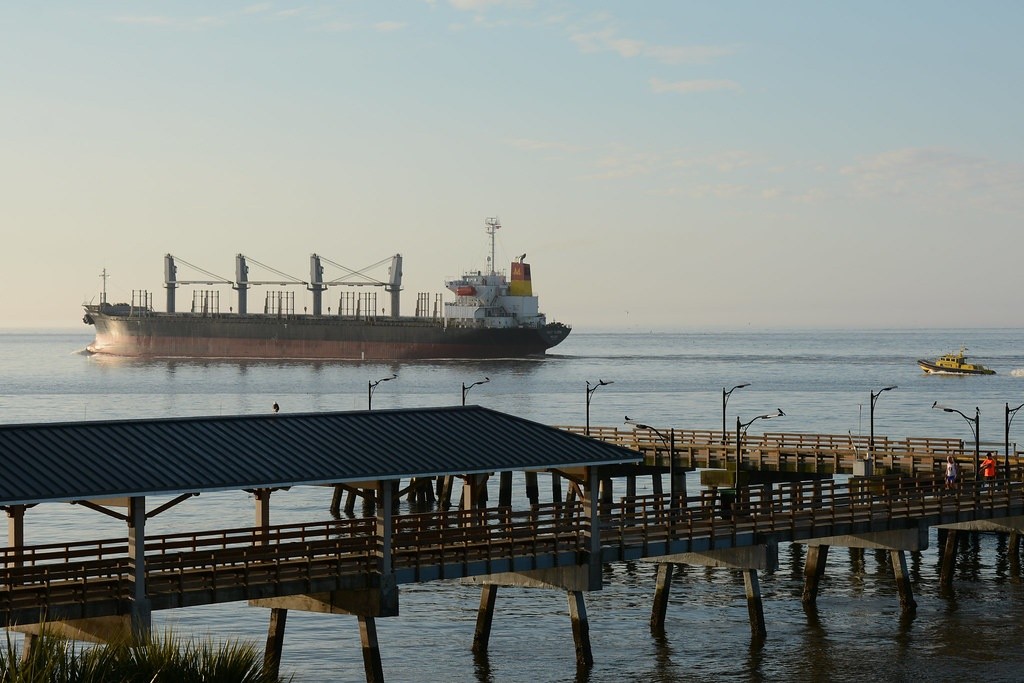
left=870, top=386, right=898, bottom=456
left=723, top=384, right=752, bottom=456
left=624, top=421, right=677, bottom=535
left=368, top=377, right=396, bottom=410
left=585, top=380, right=614, bottom=436
left=462, top=380, right=490, bottom=405
left=934, top=406, right=981, bottom=498
left=737, top=414, right=783, bottom=504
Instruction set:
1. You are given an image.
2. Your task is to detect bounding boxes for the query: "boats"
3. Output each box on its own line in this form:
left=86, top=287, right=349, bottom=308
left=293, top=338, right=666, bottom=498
left=82, top=217, right=572, bottom=359
left=916, top=344, right=996, bottom=374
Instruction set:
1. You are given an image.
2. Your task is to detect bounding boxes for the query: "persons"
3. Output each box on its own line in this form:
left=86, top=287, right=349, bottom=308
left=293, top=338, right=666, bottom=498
left=944, top=456, right=957, bottom=496
left=980, top=452, right=996, bottom=493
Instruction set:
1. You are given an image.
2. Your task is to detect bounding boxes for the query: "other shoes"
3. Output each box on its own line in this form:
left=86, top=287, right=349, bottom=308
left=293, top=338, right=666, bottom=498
left=984, top=497, right=993, bottom=502
left=945, top=495, right=956, bottom=499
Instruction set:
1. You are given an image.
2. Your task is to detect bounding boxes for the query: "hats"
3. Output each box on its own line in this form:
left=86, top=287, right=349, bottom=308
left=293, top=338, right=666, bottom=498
left=985, top=452, right=992, bottom=457
